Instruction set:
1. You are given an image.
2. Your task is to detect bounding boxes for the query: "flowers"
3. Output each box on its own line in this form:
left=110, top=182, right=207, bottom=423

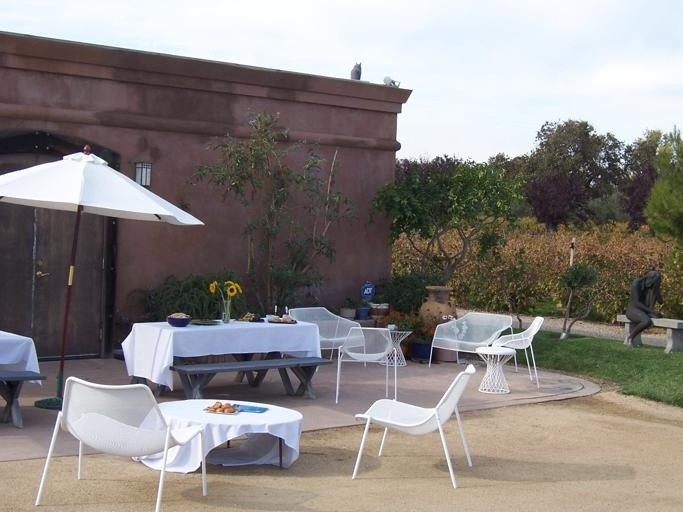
left=441, top=314, right=468, bottom=339
left=209, top=280, right=243, bottom=319
left=412, top=317, right=438, bottom=342
left=380, top=312, right=416, bottom=337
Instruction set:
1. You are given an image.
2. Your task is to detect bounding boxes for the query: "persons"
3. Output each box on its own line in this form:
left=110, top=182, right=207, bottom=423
left=626, top=270, right=663, bottom=342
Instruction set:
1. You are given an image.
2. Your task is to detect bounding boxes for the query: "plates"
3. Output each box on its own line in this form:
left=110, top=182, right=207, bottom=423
left=190, top=319, right=217, bottom=325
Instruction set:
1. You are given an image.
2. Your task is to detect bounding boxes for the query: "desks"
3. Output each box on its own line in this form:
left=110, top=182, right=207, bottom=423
left=0, top=329, right=43, bottom=388
left=120, top=319, right=322, bottom=400
left=131, top=399, right=304, bottom=475
left=376, top=330, right=414, bottom=367
left=475, top=347, right=515, bottom=395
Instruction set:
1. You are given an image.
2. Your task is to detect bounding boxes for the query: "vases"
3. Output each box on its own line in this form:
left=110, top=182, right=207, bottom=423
left=409, top=335, right=431, bottom=362
left=416, top=286, right=456, bottom=328
left=220, top=301, right=232, bottom=322
left=434, top=340, right=457, bottom=362
left=386, top=341, right=409, bottom=362
left=339, top=302, right=388, bottom=321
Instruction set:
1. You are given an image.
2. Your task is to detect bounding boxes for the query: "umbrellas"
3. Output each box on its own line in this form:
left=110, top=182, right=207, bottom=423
left=0, top=143, right=206, bottom=397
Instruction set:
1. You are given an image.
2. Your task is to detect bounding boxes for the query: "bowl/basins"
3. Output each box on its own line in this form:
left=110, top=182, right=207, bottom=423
left=167, top=315, right=191, bottom=326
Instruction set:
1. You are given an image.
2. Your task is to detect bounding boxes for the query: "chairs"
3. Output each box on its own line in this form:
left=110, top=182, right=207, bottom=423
left=349, top=364, right=480, bottom=489
left=335, top=326, right=397, bottom=405
left=287, top=306, right=367, bottom=375
left=427, top=312, right=519, bottom=374
left=486, top=315, right=544, bottom=390
left=34, top=374, right=209, bottom=512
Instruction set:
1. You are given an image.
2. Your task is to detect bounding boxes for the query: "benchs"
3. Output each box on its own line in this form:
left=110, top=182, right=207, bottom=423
left=112, top=350, right=255, bottom=387
left=614, top=313, right=682, bottom=357
left=169, top=355, right=333, bottom=400
left=0, top=370, right=46, bottom=430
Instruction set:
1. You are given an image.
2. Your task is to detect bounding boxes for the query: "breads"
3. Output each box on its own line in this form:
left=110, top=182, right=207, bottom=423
left=209, top=402, right=233, bottom=413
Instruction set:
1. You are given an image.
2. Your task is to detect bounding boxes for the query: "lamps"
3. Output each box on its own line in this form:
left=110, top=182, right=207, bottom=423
left=134, top=159, right=152, bottom=188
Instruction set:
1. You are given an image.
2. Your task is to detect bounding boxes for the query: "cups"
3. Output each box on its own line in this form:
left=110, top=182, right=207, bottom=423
left=269, top=315, right=291, bottom=322
